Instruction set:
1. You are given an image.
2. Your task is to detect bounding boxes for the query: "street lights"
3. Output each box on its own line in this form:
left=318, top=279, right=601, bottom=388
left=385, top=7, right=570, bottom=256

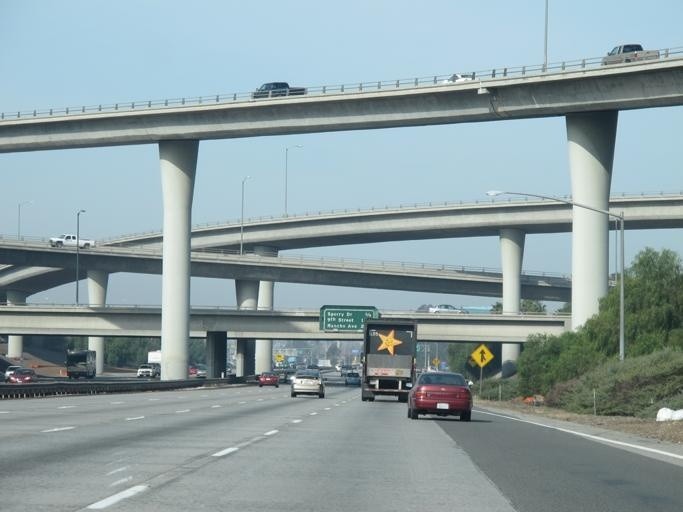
left=284, top=143, right=304, bottom=218
left=18, top=200, right=35, bottom=241
left=240, top=175, right=251, bottom=256
left=486, top=190, right=625, bottom=361
left=76, top=208, right=87, bottom=306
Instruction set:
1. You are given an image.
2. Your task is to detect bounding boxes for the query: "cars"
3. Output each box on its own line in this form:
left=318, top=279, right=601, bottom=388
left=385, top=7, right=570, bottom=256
left=344, top=371, right=362, bottom=387
left=188, top=365, right=199, bottom=375
left=258, top=372, right=280, bottom=388
left=196, top=367, right=208, bottom=378
left=225, top=359, right=235, bottom=375
left=3, top=366, right=23, bottom=382
left=290, top=368, right=328, bottom=398
left=272, top=369, right=287, bottom=383
left=428, top=304, right=469, bottom=315
left=286, top=370, right=298, bottom=385
left=608, top=273, right=632, bottom=284
left=146, top=363, right=161, bottom=377
left=8, top=369, right=38, bottom=384
left=416, top=366, right=440, bottom=379
left=405, top=371, right=474, bottom=421
left=137, top=364, right=156, bottom=378
left=272, top=355, right=364, bottom=377
left=442, top=74, right=479, bottom=85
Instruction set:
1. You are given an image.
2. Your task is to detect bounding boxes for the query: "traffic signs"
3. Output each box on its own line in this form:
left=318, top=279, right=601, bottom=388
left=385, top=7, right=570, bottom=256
left=323, top=309, right=375, bottom=332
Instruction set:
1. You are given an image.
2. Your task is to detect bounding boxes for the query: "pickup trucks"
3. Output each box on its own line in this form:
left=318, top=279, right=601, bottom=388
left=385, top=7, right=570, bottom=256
left=250, top=82, right=308, bottom=99
left=601, top=44, right=660, bottom=65
left=49, top=233, right=96, bottom=250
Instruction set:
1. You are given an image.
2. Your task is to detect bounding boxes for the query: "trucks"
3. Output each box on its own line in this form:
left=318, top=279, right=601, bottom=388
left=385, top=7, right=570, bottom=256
left=361, top=317, right=417, bottom=403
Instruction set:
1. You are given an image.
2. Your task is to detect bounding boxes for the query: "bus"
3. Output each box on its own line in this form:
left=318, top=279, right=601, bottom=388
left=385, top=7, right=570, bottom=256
left=64, top=350, right=96, bottom=380
